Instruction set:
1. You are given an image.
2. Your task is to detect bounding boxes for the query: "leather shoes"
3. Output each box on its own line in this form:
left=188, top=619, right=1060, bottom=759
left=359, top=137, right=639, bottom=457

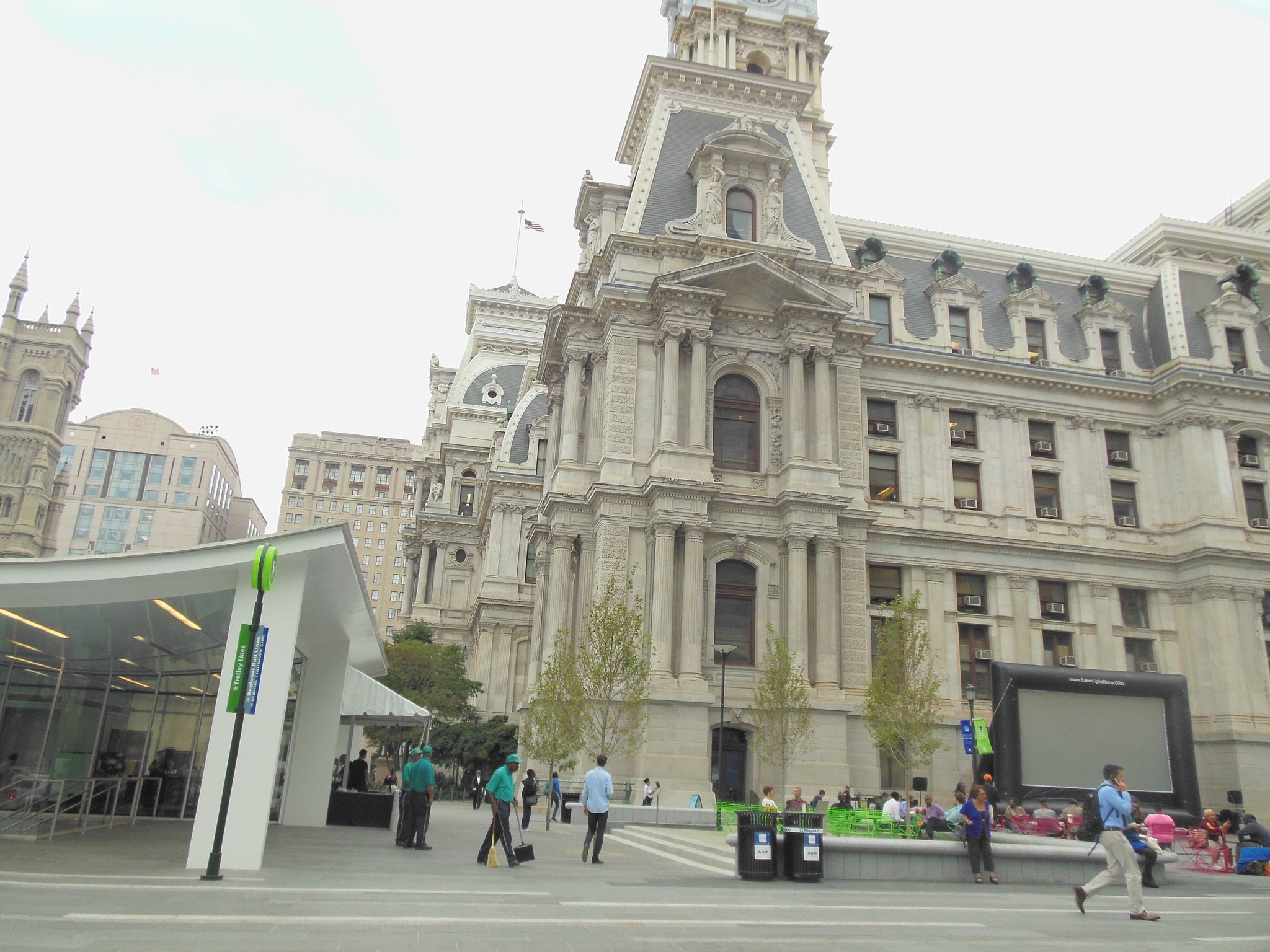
left=1130, top=910, right=1160, bottom=921
left=1075, top=886, right=1085, bottom=913
left=921, top=834, right=931, bottom=840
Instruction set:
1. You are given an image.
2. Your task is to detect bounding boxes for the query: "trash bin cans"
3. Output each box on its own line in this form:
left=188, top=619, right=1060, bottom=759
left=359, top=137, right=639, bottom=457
left=734, top=810, right=779, bottom=880
left=781, top=812, right=826, bottom=883
left=560, top=792, right=581, bottom=824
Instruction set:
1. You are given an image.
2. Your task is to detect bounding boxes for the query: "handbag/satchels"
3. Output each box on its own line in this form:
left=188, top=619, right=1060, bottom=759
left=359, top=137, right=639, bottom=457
left=953, top=814, right=967, bottom=838
left=526, top=796, right=537, bottom=807
left=1189, top=829, right=1208, bottom=848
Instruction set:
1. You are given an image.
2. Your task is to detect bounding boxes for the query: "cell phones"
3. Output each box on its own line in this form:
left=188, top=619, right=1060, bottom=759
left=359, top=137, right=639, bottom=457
left=1110, top=776, right=1118, bottom=785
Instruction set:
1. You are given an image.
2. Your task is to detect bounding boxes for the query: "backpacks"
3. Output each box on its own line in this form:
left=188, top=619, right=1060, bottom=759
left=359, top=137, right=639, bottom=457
left=543, top=778, right=557, bottom=794
left=1083, top=784, right=1115, bottom=836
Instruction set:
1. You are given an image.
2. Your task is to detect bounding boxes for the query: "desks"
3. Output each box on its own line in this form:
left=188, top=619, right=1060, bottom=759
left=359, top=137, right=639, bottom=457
left=1208, top=832, right=1238, bottom=874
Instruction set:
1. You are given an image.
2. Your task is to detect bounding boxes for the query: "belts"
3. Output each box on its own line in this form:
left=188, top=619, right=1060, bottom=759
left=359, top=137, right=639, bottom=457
left=1102, top=827, right=1120, bottom=831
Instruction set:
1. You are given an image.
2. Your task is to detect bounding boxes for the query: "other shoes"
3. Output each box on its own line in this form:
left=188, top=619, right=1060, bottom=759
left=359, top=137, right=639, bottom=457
left=976, top=877, right=983, bottom=884
left=592, top=860, right=604, bottom=864
left=990, top=877, right=999, bottom=884
left=509, top=859, right=519, bottom=867
left=1210, top=864, right=1222, bottom=871
left=521, top=828, right=529, bottom=831
left=477, top=857, right=488, bottom=864
left=550, top=817, right=557, bottom=822
left=477, top=807, right=479, bottom=810
left=396, top=840, right=432, bottom=850
left=1141, top=877, right=1159, bottom=888
left=582, top=844, right=589, bottom=863
left=473, top=808, right=476, bottom=810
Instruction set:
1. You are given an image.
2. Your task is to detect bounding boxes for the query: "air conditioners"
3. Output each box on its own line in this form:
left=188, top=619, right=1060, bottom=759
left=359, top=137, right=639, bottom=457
left=875, top=424, right=888, bottom=433
left=1111, top=451, right=1128, bottom=462
left=975, top=650, right=993, bottom=660
left=1041, top=507, right=1058, bottom=518
left=1047, top=603, right=1064, bottom=613
left=958, top=348, right=973, bottom=356
left=1237, top=368, right=1254, bottom=377
left=1141, top=663, right=1159, bottom=673
left=1059, top=656, right=1077, bottom=666
left=965, top=596, right=981, bottom=606
left=951, top=429, right=965, bottom=441
left=961, top=499, right=977, bottom=510
left=1109, top=370, right=1127, bottom=379
left=1251, top=518, right=1269, bottom=528
left=1242, top=455, right=1259, bottom=466
left=1035, top=441, right=1052, bottom=453
left=1035, top=360, right=1050, bottom=367
left=1119, top=517, right=1135, bottom=527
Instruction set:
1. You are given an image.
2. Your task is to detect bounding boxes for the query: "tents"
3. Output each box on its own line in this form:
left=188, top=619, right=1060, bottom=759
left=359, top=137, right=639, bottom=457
left=335, top=664, right=433, bottom=789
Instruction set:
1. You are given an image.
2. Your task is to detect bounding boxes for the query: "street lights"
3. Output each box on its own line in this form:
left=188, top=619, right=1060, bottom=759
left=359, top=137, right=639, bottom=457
left=710, top=644, right=738, bottom=830
left=965, top=683, right=977, bottom=779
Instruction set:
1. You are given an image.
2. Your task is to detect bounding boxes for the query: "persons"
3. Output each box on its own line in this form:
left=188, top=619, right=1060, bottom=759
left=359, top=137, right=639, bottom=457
left=477, top=754, right=524, bottom=867
left=582, top=754, right=614, bottom=864
left=403, top=746, right=435, bottom=850
left=959, top=785, right=998, bottom=885
left=1072, top=764, right=1160, bottom=921
left=334, top=749, right=1270, bottom=876
left=395, top=748, right=423, bottom=846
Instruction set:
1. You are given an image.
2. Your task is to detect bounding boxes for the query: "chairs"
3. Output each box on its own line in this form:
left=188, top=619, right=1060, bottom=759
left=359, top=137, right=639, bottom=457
left=992, top=814, right=1226, bottom=874
left=715, top=801, right=960, bottom=840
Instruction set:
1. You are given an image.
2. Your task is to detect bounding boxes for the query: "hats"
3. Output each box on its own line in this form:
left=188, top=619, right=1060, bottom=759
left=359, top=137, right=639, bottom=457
left=410, top=748, right=422, bottom=756
left=506, top=755, right=523, bottom=763
left=423, top=746, right=433, bottom=754
left=983, top=774, right=992, bottom=781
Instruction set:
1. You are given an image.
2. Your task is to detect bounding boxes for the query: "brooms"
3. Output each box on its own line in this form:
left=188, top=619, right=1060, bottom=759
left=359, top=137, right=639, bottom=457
left=486, top=798, right=500, bottom=868
